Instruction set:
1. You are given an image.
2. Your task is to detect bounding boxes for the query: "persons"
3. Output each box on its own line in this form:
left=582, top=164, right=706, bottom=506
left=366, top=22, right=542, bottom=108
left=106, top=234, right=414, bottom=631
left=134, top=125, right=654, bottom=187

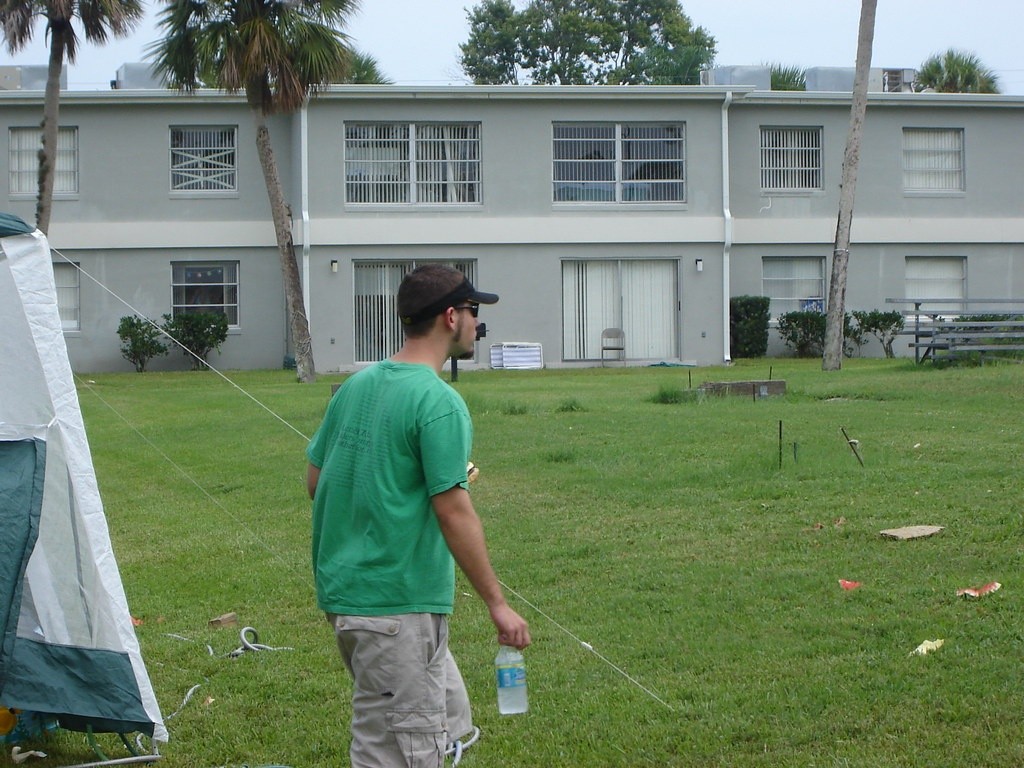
left=305, top=264, right=531, bottom=768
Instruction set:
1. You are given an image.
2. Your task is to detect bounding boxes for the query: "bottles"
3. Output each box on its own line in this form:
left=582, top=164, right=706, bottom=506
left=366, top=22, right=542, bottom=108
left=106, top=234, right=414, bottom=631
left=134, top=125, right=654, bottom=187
left=494, top=645, right=529, bottom=715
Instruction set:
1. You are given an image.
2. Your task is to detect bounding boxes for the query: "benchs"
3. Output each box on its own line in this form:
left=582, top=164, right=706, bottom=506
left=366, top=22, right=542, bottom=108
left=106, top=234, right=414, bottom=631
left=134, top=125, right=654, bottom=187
left=885, top=298, right=1024, bottom=368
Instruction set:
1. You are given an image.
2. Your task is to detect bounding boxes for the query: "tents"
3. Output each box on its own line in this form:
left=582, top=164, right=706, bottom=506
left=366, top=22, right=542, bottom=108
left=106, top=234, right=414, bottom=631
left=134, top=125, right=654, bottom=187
left=0, top=215, right=171, bottom=768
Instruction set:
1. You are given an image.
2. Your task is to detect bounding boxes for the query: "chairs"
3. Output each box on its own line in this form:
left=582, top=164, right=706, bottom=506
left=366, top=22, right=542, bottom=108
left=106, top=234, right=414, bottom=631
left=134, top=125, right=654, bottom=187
left=601, top=328, right=626, bottom=369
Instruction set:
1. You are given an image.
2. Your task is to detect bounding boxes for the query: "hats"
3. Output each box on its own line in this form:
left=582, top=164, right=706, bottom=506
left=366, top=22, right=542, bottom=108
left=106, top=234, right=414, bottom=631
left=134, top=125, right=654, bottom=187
left=399, top=276, right=499, bottom=324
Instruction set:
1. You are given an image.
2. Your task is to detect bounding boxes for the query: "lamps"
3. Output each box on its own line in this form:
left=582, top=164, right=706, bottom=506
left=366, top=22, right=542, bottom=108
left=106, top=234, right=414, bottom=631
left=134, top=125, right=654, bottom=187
left=695, top=258, right=703, bottom=272
left=331, top=259, right=337, bottom=273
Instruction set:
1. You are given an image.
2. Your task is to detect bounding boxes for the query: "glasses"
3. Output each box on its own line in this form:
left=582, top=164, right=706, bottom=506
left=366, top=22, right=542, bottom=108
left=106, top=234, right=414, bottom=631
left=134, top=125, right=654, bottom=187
left=439, top=303, right=479, bottom=318
